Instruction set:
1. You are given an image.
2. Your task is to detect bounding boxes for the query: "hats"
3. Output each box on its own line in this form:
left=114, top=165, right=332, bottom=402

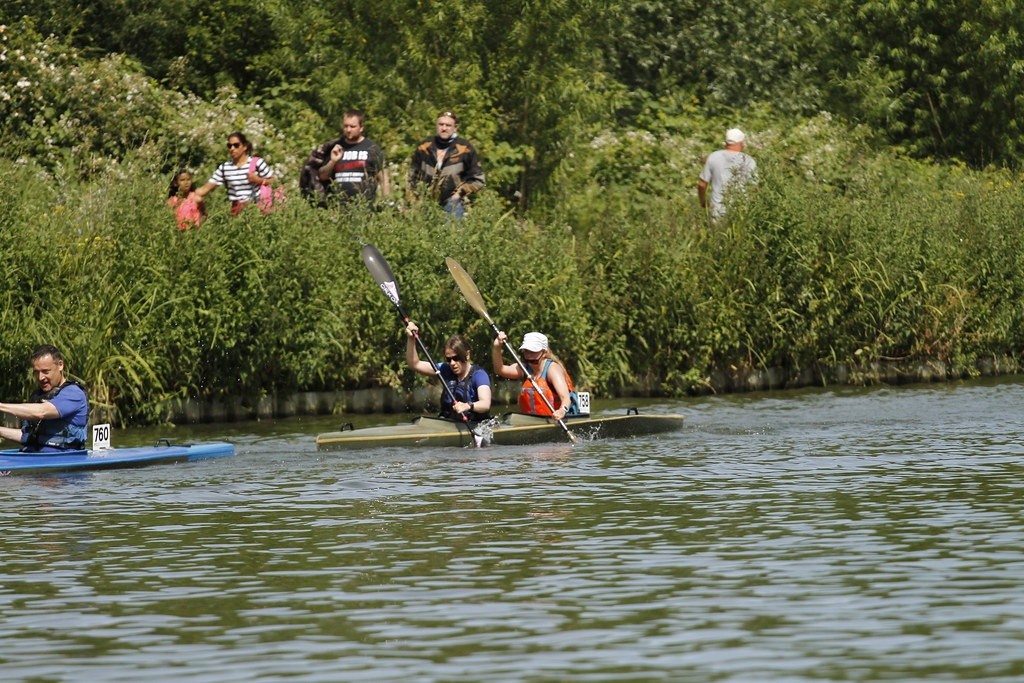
left=437, top=111, right=456, bottom=119
left=725, top=128, right=744, bottom=144
left=519, top=332, right=548, bottom=352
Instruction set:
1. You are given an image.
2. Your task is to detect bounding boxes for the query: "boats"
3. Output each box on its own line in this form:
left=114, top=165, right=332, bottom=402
left=315, top=406, right=685, bottom=451
left=0, top=439, right=237, bottom=476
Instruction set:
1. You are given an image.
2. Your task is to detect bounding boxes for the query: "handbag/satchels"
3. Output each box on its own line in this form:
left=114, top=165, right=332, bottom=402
left=249, top=157, right=285, bottom=215
left=300, top=141, right=332, bottom=210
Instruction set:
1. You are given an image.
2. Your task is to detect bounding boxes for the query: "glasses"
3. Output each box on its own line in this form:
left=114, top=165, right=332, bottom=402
left=446, top=355, right=462, bottom=361
left=227, top=142, right=240, bottom=148
left=523, top=352, right=544, bottom=364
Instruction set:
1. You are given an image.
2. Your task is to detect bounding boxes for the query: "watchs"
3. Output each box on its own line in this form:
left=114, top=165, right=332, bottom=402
left=560, top=405, right=569, bottom=412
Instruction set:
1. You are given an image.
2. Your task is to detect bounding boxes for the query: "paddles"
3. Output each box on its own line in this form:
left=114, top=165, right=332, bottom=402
left=444, top=255, right=577, bottom=444
left=360, top=243, right=480, bottom=447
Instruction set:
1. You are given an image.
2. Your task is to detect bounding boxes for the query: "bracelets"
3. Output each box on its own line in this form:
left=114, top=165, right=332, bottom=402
left=468, top=402, right=474, bottom=412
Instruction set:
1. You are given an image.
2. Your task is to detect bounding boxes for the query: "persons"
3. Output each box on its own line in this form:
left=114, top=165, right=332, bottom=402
left=493, top=331, right=578, bottom=421
left=195, top=132, right=274, bottom=216
left=0, top=344, right=90, bottom=451
left=406, top=111, right=485, bottom=217
left=319, top=110, right=390, bottom=212
left=406, top=323, right=491, bottom=422
left=698, top=128, right=757, bottom=223
left=166, top=169, right=207, bottom=231
